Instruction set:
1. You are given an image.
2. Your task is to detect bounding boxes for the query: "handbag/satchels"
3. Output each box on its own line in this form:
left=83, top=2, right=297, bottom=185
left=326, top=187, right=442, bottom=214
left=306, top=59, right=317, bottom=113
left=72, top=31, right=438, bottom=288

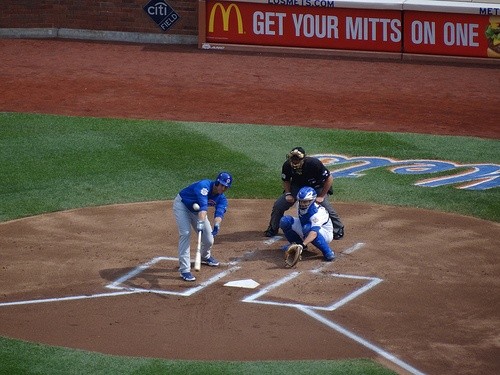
left=322, top=182, right=333, bottom=195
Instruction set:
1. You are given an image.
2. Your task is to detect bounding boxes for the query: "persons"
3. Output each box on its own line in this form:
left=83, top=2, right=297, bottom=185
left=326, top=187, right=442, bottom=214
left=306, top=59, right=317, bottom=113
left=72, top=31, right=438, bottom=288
left=173, top=172, right=232, bottom=281
left=280, top=187, right=335, bottom=260
left=259, top=147, right=344, bottom=240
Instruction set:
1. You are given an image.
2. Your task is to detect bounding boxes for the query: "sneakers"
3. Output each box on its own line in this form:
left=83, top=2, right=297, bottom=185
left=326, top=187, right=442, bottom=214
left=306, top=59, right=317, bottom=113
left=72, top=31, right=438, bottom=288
left=179, top=271, right=196, bottom=281
left=326, top=248, right=336, bottom=260
left=201, top=257, right=219, bottom=266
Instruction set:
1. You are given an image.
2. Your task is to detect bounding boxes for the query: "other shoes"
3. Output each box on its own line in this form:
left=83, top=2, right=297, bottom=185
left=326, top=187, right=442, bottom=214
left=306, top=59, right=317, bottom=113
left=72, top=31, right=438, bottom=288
left=264, top=226, right=278, bottom=236
left=334, top=227, right=343, bottom=239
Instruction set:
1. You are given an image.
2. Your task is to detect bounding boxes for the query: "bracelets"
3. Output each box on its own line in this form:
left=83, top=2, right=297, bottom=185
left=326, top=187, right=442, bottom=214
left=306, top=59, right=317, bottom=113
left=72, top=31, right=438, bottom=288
left=285, top=193, right=290, bottom=196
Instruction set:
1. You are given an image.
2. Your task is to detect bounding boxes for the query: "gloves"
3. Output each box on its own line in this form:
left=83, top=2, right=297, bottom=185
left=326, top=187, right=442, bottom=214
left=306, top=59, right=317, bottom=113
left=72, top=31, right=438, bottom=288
left=196, top=220, right=203, bottom=233
left=211, top=222, right=219, bottom=237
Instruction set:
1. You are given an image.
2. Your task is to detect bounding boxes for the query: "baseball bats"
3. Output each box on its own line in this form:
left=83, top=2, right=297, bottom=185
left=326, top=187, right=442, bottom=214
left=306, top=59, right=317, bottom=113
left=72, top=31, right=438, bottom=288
left=194, top=231, right=202, bottom=273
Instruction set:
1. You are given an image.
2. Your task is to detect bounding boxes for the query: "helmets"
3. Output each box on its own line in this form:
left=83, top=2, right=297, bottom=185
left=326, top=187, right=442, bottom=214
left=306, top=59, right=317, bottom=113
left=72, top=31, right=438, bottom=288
left=215, top=172, right=232, bottom=187
left=296, top=187, right=317, bottom=217
left=288, top=146, right=305, bottom=170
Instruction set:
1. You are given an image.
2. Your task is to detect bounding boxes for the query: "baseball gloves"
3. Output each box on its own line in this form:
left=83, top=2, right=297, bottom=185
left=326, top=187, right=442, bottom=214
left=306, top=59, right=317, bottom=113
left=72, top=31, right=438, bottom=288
left=284, top=243, right=303, bottom=267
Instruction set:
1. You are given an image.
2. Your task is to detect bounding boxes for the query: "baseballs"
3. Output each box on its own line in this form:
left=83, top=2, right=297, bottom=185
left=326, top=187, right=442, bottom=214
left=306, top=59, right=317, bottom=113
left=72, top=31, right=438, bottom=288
left=192, top=203, right=200, bottom=210
